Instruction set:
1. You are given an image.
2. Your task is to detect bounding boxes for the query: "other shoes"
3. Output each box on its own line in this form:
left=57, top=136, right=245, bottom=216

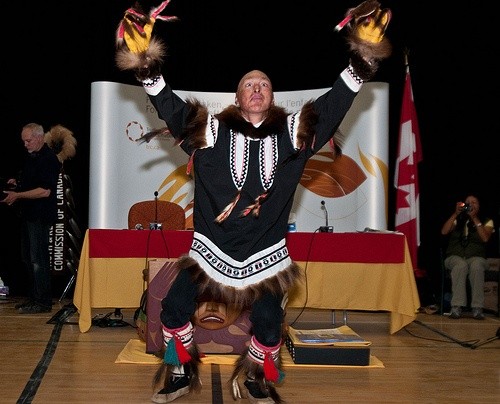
left=15, top=299, right=52, bottom=314
left=472, top=309, right=485, bottom=319
left=449, top=308, right=461, bottom=318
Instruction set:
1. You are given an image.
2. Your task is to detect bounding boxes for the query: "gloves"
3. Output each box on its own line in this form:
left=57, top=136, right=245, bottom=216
left=346, top=0, right=392, bottom=59
left=117, top=2, right=165, bottom=70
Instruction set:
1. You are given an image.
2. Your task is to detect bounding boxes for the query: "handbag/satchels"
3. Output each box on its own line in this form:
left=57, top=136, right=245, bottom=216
left=461, top=237, right=479, bottom=258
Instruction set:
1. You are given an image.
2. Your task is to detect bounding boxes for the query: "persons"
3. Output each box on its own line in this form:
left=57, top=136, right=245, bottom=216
left=0, top=123, right=61, bottom=313
left=441, top=193, right=494, bottom=320
left=115, top=0, right=393, bottom=404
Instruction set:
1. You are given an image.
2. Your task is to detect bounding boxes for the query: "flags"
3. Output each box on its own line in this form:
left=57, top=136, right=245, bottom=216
left=393, top=72, right=422, bottom=276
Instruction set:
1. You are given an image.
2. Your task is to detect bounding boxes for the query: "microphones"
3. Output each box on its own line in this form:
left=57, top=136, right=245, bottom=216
left=320, top=201, right=334, bottom=233
left=149, top=191, right=162, bottom=229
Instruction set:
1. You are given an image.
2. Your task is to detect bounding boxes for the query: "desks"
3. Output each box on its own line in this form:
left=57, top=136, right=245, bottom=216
left=72, top=229, right=421, bottom=334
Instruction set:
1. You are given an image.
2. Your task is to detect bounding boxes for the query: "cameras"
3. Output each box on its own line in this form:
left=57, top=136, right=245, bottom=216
left=464, top=204, right=473, bottom=212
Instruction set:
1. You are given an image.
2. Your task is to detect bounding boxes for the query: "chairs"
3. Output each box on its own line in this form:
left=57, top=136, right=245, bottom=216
left=438, top=247, right=471, bottom=315
left=128, top=200, right=186, bottom=230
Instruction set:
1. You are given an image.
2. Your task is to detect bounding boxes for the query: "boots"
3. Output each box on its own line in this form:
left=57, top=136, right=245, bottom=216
left=151, top=322, right=202, bottom=404
left=229, top=335, right=285, bottom=404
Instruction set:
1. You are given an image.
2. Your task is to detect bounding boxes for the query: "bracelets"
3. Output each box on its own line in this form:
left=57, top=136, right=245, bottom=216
left=476, top=223, right=482, bottom=226
